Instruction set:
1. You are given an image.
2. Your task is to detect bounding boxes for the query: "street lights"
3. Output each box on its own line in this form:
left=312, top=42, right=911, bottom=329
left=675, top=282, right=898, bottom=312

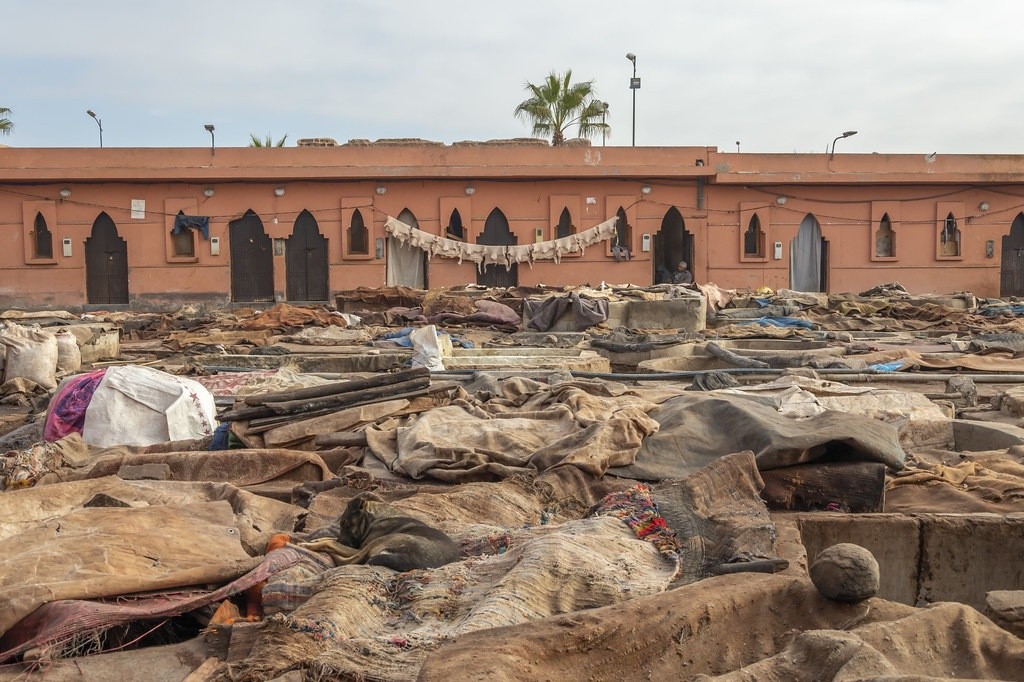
left=87, top=109, right=103, bottom=147
left=626, top=53, right=638, bottom=147
left=736, top=140, right=741, bottom=153
left=602, top=101, right=609, bottom=147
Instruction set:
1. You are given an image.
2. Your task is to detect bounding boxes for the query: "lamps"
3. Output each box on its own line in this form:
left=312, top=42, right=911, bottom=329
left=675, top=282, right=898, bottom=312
left=59, top=186, right=73, bottom=198
left=204, top=124, right=215, bottom=156
left=375, top=185, right=388, bottom=195
left=640, top=185, right=652, bottom=195
left=274, top=185, right=287, bottom=196
left=978, top=201, right=992, bottom=211
left=202, top=188, right=215, bottom=198
left=829, top=131, right=858, bottom=161
left=464, top=185, right=476, bottom=196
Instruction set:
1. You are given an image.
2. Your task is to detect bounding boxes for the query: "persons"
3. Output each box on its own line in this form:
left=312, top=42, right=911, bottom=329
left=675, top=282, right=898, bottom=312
left=665, top=261, right=692, bottom=284
